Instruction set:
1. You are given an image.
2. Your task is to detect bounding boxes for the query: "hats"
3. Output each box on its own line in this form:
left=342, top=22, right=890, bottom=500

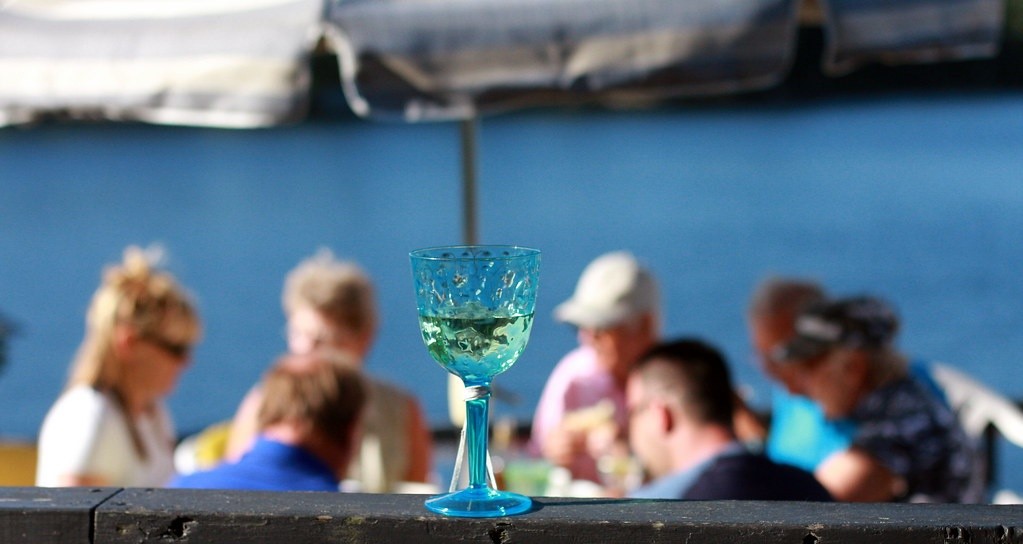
left=552, top=250, right=658, bottom=329
left=773, top=302, right=852, bottom=363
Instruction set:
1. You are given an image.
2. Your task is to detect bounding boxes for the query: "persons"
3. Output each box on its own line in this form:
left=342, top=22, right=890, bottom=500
left=529, top=251, right=765, bottom=491
left=178, top=258, right=430, bottom=485
left=753, top=278, right=1022, bottom=504
left=622, top=338, right=835, bottom=501
left=37, top=249, right=196, bottom=489
left=174, top=358, right=371, bottom=491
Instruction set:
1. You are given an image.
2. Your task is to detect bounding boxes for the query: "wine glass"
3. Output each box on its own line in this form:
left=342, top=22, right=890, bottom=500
left=408, top=243, right=544, bottom=519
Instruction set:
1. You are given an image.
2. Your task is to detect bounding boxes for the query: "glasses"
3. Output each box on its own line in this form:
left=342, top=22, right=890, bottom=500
left=137, top=328, right=190, bottom=364
